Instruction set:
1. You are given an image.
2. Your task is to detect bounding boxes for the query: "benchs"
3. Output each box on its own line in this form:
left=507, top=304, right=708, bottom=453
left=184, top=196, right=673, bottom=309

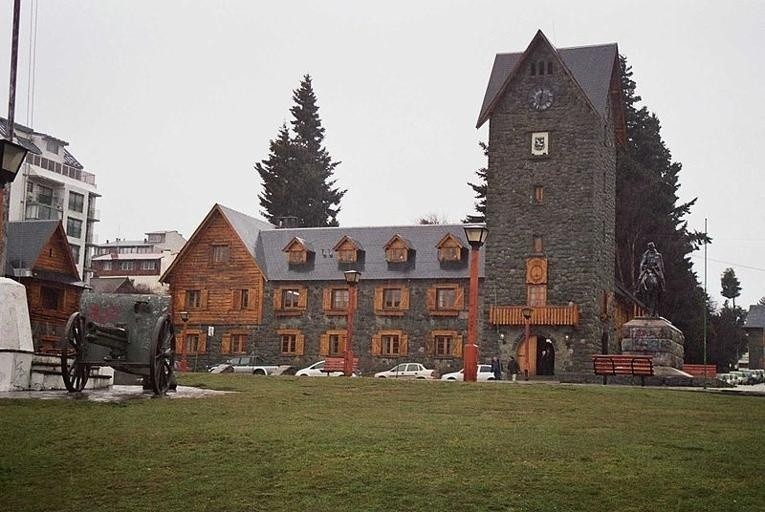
left=319, top=355, right=359, bottom=377
left=590, top=352, right=656, bottom=388
left=681, top=363, right=717, bottom=388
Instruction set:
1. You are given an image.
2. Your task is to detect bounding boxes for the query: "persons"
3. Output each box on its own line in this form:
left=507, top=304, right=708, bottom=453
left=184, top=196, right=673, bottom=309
left=491, top=357, right=502, bottom=379
left=507, top=356, right=521, bottom=381
left=539, top=350, right=547, bottom=375
left=641, top=241, right=666, bottom=291
left=545, top=350, right=554, bottom=375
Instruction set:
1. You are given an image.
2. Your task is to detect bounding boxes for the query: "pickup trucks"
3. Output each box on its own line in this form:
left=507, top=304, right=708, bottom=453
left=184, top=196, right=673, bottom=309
left=206, top=354, right=279, bottom=378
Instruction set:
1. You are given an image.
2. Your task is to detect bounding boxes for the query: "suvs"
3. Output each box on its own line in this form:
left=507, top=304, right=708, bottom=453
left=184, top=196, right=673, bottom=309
left=441, top=364, right=496, bottom=381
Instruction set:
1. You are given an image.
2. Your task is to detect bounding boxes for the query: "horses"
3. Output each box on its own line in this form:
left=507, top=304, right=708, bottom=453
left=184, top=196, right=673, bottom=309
left=641, top=263, right=665, bottom=318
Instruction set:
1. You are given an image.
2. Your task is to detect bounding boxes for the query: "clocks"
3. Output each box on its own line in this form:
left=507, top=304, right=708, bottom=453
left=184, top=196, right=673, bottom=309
left=524, top=82, right=555, bottom=112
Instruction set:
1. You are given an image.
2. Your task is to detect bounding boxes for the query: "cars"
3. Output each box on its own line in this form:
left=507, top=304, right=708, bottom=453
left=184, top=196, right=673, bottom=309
left=715, top=368, right=765, bottom=386
left=295, top=359, right=357, bottom=378
left=373, top=361, right=441, bottom=380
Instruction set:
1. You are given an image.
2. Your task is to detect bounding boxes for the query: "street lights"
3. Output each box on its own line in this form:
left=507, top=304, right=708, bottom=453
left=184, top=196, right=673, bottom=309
left=177, top=310, right=196, bottom=373
left=521, top=307, right=534, bottom=380
left=460, top=224, right=492, bottom=384
left=342, top=269, right=360, bottom=379
